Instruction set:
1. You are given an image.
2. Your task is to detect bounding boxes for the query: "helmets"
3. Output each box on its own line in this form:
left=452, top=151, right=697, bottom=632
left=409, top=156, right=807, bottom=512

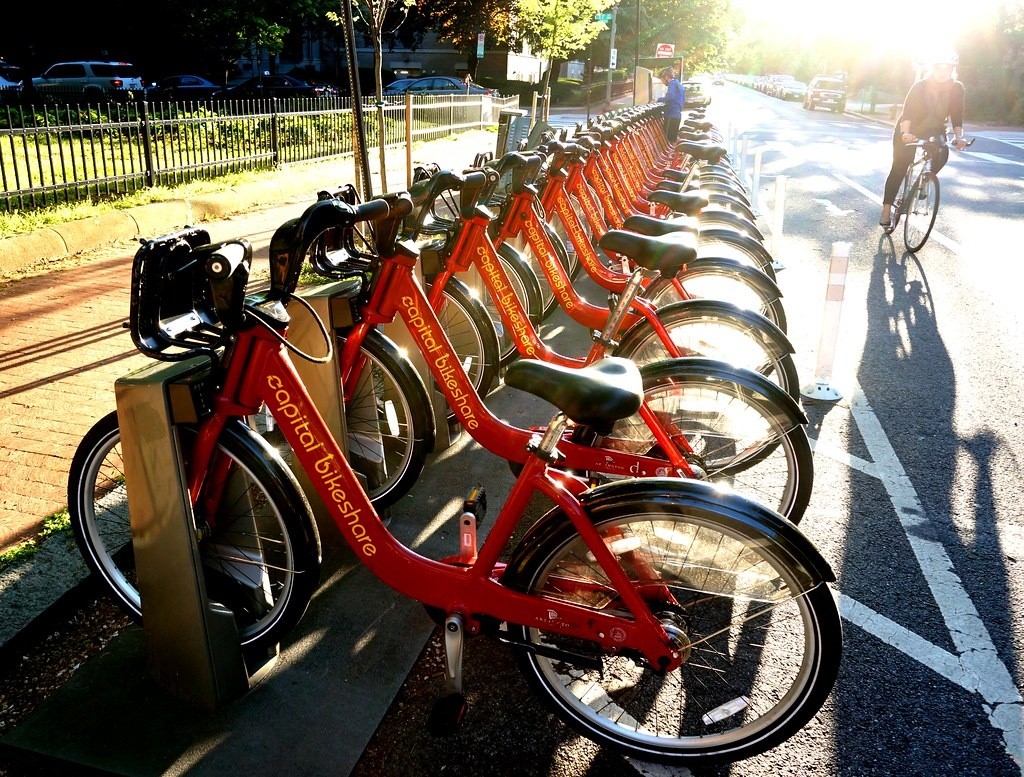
left=657, top=66, right=674, bottom=78
left=935, top=48, right=959, bottom=66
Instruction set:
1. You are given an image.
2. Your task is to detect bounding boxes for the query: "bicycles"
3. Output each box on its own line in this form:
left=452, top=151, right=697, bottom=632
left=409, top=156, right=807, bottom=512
left=314, top=190, right=817, bottom=559
left=464, top=104, right=785, bottom=377
left=407, top=164, right=800, bottom=475
left=69, top=187, right=845, bottom=763
left=882, top=137, right=974, bottom=254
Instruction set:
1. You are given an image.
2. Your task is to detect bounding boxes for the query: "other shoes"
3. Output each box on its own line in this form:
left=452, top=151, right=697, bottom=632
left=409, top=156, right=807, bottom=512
left=918, top=189, right=926, bottom=201
left=879, top=217, right=891, bottom=227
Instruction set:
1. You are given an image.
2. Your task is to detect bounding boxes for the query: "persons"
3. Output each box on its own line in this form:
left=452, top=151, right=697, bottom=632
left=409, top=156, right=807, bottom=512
left=880, top=49, right=968, bottom=225
left=653, top=66, right=684, bottom=142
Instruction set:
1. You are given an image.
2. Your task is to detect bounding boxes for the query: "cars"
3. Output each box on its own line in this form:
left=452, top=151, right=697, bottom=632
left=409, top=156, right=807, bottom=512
left=379, top=77, right=501, bottom=101
left=210, top=73, right=341, bottom=112
left=724, top=74, right=769, bottom=91
left=145, top=75, right=226, bottom=101
left=711, top=75, right=724, bottom=86
left=777, top=81, right=807, bottom=100
left=680, top=82, right=713, bottom=105
left=368, top=79, right=427, bottom=97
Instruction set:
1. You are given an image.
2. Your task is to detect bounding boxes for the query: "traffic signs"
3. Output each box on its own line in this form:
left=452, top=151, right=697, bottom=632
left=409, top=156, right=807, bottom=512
left=610, top=49, right=617, bottom=69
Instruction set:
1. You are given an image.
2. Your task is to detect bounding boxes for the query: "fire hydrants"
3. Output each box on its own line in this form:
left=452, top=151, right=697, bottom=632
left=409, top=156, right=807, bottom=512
left=890, top=104, right=898, bottom=120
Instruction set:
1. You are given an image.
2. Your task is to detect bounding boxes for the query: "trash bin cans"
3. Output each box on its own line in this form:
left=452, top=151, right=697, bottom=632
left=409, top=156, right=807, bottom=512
left=494, top=108, right=539, bottom=160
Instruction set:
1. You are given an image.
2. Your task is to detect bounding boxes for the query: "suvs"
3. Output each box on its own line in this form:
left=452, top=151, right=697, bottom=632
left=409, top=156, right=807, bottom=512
left=0, top=65, right=23, bottom=95
left=766, top=75, right=795, bottom=96
left=18, top=60, right=147, bottom=100
left=803, top=76, right=848, bottom=113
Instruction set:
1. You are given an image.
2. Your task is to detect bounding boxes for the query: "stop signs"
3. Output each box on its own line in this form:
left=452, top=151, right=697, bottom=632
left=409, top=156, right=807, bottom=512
left=655, top=43, right=675, bottom=58
